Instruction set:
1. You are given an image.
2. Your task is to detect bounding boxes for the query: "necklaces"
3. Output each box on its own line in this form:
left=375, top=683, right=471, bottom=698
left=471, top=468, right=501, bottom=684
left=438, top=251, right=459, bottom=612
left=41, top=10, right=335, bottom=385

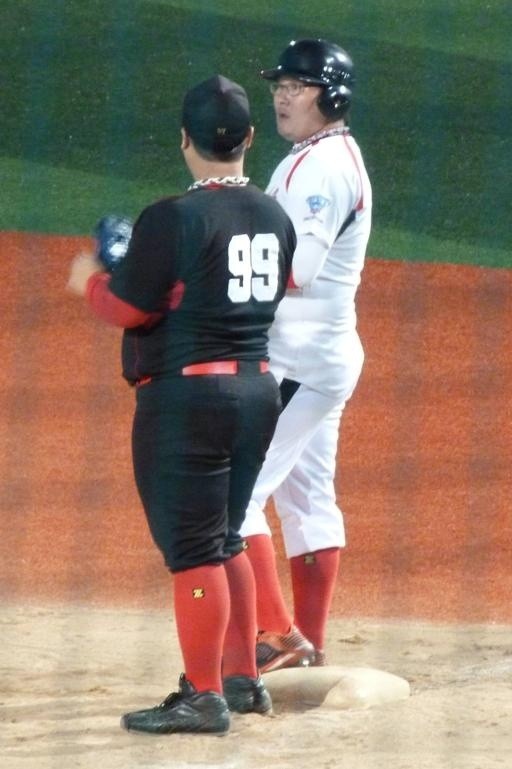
left=291, top=126, right=349, bottom=154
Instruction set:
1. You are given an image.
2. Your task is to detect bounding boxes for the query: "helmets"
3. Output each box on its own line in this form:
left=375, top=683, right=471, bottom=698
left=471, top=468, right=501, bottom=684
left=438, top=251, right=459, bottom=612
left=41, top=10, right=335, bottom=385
left=261, top=38, right=354, bottom=119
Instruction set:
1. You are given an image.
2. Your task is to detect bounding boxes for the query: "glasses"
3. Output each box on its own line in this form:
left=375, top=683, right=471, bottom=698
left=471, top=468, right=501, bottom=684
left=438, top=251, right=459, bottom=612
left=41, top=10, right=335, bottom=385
left=270, top=80, right=325, bottom=98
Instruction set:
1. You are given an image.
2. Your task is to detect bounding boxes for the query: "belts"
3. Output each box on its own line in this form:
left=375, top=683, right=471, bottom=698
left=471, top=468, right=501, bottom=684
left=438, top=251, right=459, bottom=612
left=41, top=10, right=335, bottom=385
left=136, top=359, right=271, bottom=388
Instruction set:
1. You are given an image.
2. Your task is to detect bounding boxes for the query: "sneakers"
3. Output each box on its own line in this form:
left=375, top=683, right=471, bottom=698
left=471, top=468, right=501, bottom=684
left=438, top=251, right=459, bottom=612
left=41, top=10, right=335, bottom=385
left=254, top=625, right=313, bottom=674
left=312, top=651, right=329, bottom=666
left=221, top=670, right=275, bottom=715
left=121, top=672, right=231, bottom=735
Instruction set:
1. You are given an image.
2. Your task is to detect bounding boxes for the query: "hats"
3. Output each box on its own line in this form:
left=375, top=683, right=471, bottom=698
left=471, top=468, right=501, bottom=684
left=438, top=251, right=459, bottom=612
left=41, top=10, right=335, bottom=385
left=182, top=73, right=251, bottom=149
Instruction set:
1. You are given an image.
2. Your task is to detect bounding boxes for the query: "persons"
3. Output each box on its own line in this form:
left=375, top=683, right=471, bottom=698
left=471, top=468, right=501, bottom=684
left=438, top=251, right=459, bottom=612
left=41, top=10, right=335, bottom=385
left=239, top=39, right=372, bottom=676
left=65, top=77, right=295, bottom=734
